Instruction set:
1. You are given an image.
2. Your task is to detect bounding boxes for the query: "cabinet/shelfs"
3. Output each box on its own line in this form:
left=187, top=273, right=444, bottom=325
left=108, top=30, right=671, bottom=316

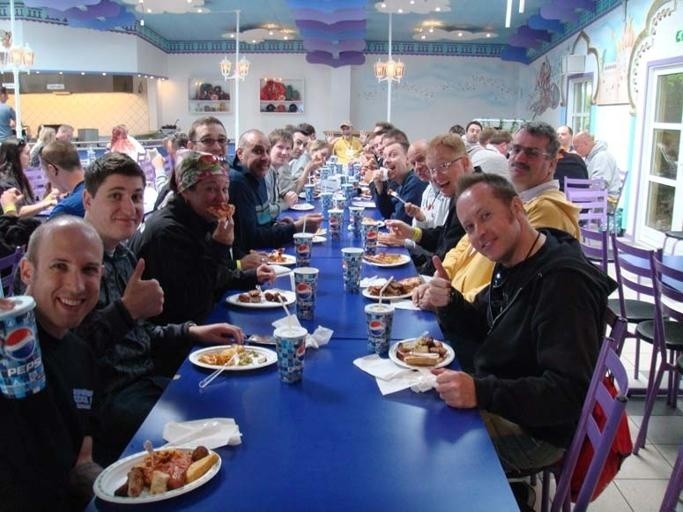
left=187, top=77, right=231, bottom=113
left=259, top=78, right=304, bottom=112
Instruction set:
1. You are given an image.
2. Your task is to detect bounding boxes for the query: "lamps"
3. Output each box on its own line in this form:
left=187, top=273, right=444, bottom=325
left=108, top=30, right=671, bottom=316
left=374, top=58, right=405, bottom=84
left=219, top=56, right=250, bottom=81
left=9, top=43, right=35, bottom=75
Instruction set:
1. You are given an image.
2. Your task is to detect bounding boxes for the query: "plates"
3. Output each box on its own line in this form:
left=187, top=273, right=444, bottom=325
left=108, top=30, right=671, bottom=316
left=187, top=250, right=296, bottom=373
left=387, top=337, right=456, bottom=371
left=362, top=252, right=415, bottom=303
left=89, top=442, right=223, bottom=505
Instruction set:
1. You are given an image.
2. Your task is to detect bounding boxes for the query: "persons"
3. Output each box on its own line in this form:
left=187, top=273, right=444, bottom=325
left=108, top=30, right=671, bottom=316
left=0, top=85, right=16, bottom=146
left=0, top=118, right=628, bottom=512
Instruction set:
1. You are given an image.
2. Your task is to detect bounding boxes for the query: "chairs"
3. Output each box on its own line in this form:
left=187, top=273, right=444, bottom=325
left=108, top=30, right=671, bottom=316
left=0, top=148, right=175, bottom=297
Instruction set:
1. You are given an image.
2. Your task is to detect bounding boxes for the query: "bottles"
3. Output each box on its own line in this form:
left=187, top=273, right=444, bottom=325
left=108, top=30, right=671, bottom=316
left=219, top=101, right=225, bottom=111
left=285, top=85, right=299, bottom=101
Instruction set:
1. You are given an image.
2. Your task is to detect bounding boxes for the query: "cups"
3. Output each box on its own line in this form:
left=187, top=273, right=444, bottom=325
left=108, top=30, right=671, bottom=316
left=291, top=153, right=381, bottom=295
left=293, top=267, right=318, bottom=324
left=87, top=151, right=96, bottom=164
left=271, top=324, right=303, bottom=384
left=204, top=106, right=209, bottom=111
left=0, top=296, right=48, bottom=401
left=364, top=303, right=394, bottom=357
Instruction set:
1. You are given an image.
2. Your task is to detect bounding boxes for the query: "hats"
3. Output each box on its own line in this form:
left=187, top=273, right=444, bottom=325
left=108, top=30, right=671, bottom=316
left=174, top=149, right=229, bottom=194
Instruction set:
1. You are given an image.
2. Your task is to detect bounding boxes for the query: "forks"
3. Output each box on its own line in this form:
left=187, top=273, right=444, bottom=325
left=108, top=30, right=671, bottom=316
left=390, top=191, right=408, bottom=205
left=199, top=352, right=241, bottom=388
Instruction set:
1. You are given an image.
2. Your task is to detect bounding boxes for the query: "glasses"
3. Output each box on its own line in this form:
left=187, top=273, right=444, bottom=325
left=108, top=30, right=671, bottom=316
left=424, top=156, right=461, bottom=178
left=191, top=136, right=231, bottom=145
left=507, top=146, right=551, bottom=159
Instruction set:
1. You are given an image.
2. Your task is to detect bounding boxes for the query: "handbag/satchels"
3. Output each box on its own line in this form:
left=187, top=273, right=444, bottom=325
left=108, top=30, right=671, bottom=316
left=556, top=377, right=632, bottom=503
